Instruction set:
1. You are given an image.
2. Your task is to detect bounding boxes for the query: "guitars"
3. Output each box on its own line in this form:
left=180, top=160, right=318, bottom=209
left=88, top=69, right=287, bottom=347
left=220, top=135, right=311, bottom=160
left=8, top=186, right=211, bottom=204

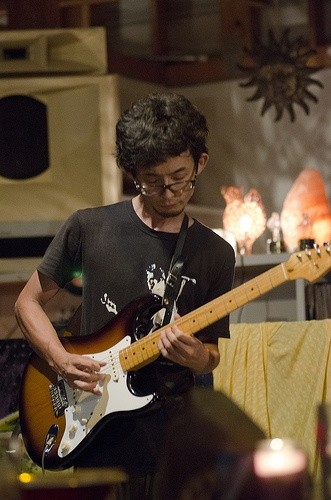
left=19, top=240, right=330, bottom=473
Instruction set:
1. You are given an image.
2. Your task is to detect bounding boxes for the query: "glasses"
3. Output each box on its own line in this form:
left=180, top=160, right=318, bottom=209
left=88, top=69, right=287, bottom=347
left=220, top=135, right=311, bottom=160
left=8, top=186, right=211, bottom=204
left=130, top=159, right=200, bottom=198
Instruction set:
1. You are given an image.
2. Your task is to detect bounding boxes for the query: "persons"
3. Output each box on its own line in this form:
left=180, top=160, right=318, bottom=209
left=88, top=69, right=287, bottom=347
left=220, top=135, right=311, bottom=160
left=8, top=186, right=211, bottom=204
left=14, top=93, right=266, bottom=500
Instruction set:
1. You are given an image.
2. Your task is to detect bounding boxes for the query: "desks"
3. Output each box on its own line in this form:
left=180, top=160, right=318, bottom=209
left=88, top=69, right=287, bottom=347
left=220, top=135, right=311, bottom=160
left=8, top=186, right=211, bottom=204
left=213, top=318, right=331, bottom=500
